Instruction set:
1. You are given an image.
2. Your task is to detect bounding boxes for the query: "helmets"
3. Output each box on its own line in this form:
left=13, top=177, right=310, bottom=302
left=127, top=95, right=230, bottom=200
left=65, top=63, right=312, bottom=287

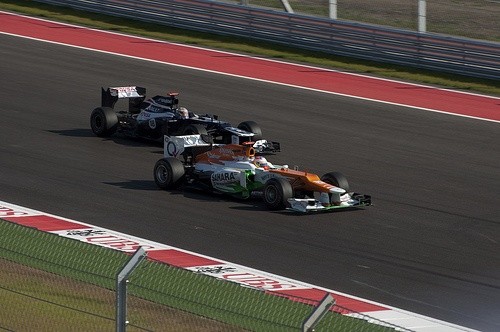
left=177, top=107, right=188, bottom=119
left=256, top=155, right=268, bottom=168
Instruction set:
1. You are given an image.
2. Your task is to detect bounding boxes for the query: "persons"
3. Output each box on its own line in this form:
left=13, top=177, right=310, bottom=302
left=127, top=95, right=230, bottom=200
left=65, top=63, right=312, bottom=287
left=255, top=156, right=269, bottom=171
left=177, top=107, right=189, bottom=119
